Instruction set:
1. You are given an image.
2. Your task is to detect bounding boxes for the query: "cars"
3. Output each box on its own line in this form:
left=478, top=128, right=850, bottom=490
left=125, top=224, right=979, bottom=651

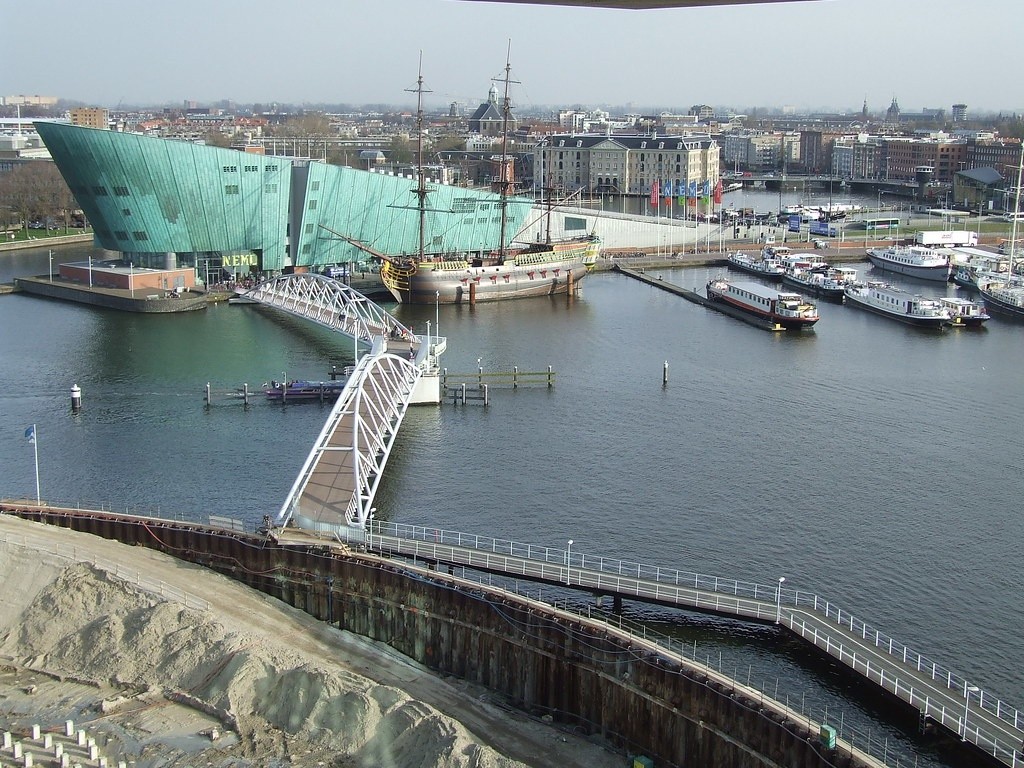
left=671, top=251, right=683, bottom=259
left=22, top=218, right=92, bottom=230
left=814, top=241, right=830, bottom=250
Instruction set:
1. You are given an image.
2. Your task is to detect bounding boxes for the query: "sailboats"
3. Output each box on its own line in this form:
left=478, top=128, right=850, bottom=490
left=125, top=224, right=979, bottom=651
left=316, top=40, right=603, bottom=305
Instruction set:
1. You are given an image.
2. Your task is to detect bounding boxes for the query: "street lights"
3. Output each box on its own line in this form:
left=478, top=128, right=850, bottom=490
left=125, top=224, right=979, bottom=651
left=567, top=540, right=574, bottom=583
left=927, top=159, right=934, bottom=166
left=776, top=577, right=786, bottom=622
left=370, top=507, right=376, bottom=550
left=961, top=686, right=980, bottom=742
left=885, top=157, right=891, bottom=181
left=958, top=162, right=965, bottom=172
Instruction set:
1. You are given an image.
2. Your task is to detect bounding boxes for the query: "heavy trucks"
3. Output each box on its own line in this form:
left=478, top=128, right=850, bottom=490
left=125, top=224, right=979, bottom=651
left=736, top=172, right=752, bottom=177
left=917, top=231, right=978, bottom=249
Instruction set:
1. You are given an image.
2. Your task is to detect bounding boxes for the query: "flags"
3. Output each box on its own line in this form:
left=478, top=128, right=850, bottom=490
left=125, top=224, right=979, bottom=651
left=23, top=427, right=36, bottom=444
left=688, top=181, right=697, bottom=206
left=714, top=180, right=722, bottom=204
left=663, top=181, right=672, bottom=206
left=677, top=180, right=686, bottom=206
left=701, top=180, right=709, bottom=204
left=650, top=182, right=658, bottom=206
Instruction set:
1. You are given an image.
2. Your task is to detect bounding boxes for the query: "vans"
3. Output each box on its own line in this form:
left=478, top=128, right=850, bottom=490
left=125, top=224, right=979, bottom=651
left=761, top=173, right=773, bottom=178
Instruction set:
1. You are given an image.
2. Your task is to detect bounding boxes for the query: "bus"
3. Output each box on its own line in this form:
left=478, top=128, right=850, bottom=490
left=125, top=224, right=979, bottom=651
left=1002, top=212, right=1024, bottom=222
left=862, top=218, right=900, bottom=230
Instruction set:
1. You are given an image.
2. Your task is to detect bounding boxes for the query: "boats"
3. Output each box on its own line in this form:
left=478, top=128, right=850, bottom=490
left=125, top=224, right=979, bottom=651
left=707, top=273, right=819, bottom=329
left=728, top=144, right=1024, bottom=328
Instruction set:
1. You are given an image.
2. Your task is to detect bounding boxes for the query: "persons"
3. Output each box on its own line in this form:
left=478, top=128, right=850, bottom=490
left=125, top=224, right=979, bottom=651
left=601, top=249, right=644, bottom=259
left=907, top=216, right=912, bottom=225
left=674, top=250, right=678, bottom=256
